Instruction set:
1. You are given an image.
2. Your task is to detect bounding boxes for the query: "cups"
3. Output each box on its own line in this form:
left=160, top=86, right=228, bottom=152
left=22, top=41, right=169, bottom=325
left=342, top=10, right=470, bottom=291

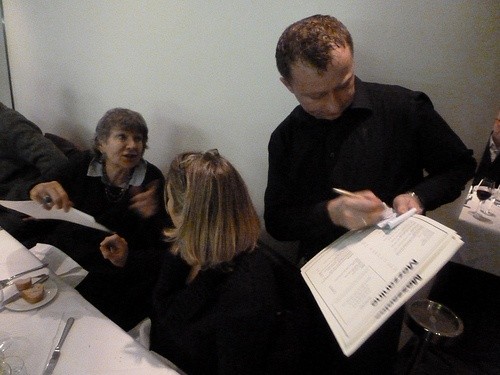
left=0, top=355, right=27, bottom=374
left=494, top=185, right=500, bottom=207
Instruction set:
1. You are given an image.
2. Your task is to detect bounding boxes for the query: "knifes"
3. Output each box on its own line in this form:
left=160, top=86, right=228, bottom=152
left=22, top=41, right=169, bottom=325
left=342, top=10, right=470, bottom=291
left=0, top=275, right=50, bottom=306
left=43, top=317, right=74, bottom=375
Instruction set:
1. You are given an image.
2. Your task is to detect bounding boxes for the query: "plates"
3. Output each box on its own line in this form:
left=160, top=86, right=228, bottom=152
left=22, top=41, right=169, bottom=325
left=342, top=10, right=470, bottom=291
left=2, top=278, right=58, bottom=311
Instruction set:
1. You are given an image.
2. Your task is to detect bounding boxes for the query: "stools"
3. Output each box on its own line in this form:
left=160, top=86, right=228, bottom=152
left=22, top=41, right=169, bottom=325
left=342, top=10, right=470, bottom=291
left=403, top=298, right=464, bottom=375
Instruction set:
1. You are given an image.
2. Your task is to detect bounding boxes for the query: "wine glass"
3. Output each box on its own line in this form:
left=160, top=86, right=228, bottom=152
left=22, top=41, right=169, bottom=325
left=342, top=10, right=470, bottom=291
left=0, top=339, right=11, bottom=375
left=468, top=177, right=495, bottom=221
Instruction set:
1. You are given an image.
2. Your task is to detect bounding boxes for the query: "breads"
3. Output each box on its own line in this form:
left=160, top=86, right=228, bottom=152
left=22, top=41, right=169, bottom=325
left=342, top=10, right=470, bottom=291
left=14, top=277, right=45, bottom=303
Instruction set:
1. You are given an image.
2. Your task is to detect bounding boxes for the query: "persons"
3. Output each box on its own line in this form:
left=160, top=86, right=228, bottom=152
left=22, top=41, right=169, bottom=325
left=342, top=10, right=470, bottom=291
left=30, top=108, right=174, bottom=311
left=0, top=103, right=71, bottom=240
left=264, top=14, right=477, bottom=360
left=472, top=112, right=500, bottom=189
left=99, top=148, right=292, bottom=375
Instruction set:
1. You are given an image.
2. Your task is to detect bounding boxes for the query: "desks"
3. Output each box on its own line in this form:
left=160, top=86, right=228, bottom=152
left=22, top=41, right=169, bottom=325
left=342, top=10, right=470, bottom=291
left=425, top=185, right=500, bottom=277
left=0, top=229, right=186, bottom=375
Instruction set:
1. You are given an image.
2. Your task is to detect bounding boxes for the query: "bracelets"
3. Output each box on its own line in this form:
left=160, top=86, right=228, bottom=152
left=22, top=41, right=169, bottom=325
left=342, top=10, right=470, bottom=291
left=408, top=191, right=420, bottom=201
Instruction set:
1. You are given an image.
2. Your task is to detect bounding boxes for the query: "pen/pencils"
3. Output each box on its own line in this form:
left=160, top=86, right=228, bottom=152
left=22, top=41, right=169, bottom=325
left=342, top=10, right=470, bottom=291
left=332, top=187, right=371, bottom=200
